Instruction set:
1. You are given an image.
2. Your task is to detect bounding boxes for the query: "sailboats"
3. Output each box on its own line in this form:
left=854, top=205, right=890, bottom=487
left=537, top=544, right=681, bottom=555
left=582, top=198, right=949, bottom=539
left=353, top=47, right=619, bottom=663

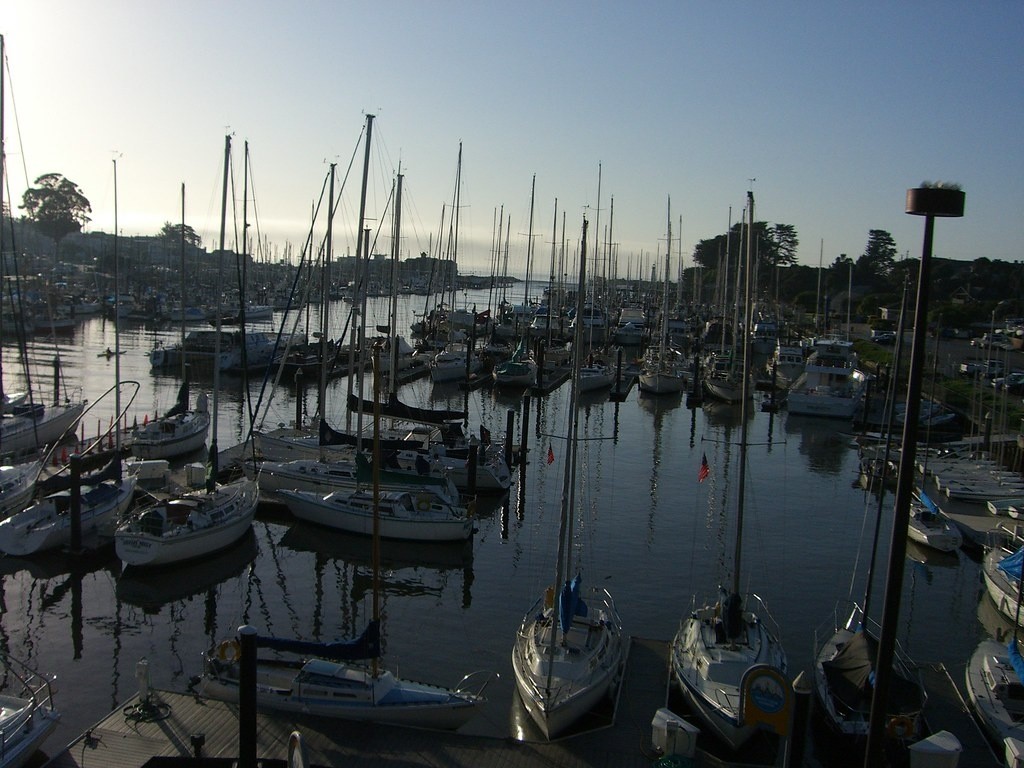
left=0, top=34, right=1024, bottom=768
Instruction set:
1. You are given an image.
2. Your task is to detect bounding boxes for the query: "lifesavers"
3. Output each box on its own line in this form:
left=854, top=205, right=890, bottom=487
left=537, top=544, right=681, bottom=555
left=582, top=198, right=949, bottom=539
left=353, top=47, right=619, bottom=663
left=219, top=639, right=239, bottom=664
left=417, top=500, right=430, bottom=513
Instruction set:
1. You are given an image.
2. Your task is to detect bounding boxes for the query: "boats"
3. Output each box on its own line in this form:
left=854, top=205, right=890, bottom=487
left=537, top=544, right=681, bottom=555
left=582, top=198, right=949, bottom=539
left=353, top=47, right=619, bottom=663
left=197, top=639, right=502, bottom=731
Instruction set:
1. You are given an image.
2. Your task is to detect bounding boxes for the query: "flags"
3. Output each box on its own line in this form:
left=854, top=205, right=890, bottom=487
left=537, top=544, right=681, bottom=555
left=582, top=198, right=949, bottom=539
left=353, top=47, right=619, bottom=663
left=697, top=452, right=710, bottom=483
left=547, top=443, right=554, bottom=465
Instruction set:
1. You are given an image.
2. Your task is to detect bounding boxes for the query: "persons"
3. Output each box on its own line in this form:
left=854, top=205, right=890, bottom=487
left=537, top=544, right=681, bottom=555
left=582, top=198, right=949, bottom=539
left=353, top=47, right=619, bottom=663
left=106, top=348, right=112, bottom=354
